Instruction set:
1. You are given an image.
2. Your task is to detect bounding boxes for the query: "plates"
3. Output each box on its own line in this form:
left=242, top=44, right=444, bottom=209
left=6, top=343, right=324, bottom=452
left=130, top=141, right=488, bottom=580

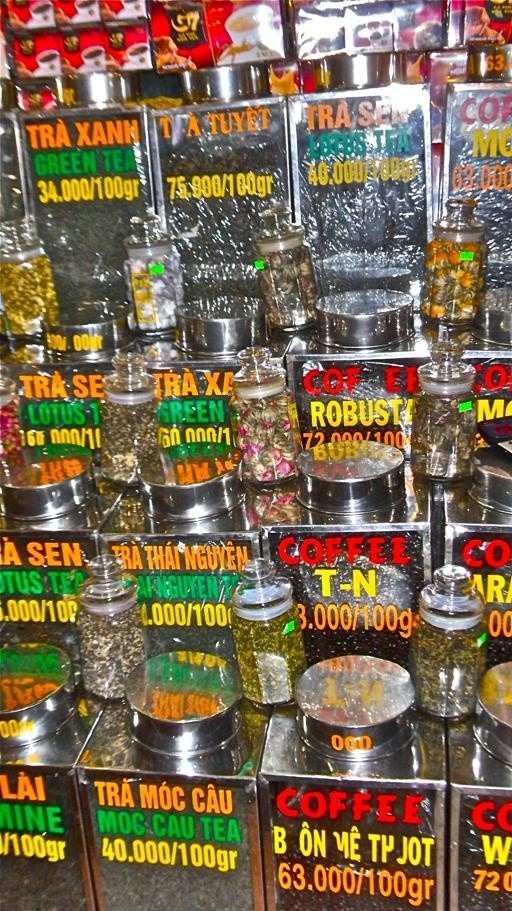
left=412, top=20, right=442, bottom=51
left=25, top=18, right=54, bottom=28
left=216, top=41, right=285, bottom=65
left=118, top=8, right=146, bottom=19
left=123, top=63, right=152, bottom=71
left=34, top=67, right=60, bottom=76
left=71, top=13, right=101, bottom=24
left=79, top=64, right=94, bottom=72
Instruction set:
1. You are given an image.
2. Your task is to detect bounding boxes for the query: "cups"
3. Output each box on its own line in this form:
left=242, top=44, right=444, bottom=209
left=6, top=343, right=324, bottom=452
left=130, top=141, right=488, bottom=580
left=225, top=6, right=284, bottom=48
left=120, top=0, right=146, bottom=14
left=74, top=1, right=99, bottom=18
left=80, top=46, right=106, bottom=72
left=370, top=26, right=393, bottom=51
left=125, top=43, right=151, bottom=69
left=29, top=1, right=53, bottom=24
left=36, top=51, right=61, bottom=72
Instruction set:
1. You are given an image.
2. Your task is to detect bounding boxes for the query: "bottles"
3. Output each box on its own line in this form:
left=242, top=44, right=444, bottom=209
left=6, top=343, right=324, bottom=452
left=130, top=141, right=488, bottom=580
left=257, top=327, right=317, bottom=358
left=120, top=216, right=186, bottom=338
left=129, top=332, right=185, bottom=367
left=412, top=476, right=473, bottom=527
left=10, top=343, right=58, bottom=375
left=0, top=360, right=20, bottom=471
left=420, top=322, right=481, bottom=353
left=73, top=553, right=147, bottom=704
left=411, top=722, right=478, bottom=783
left=230, top=558, right=310, bottom=710
left=416, top=197, right=485, bottom=326
left=0, top=216, right=58, bottom=350
left=408, top=568, right=486, bottom=726
left=247, top=208, right=317, bottom=332
left=231, top=346, right=299, bottom=485
left=239, top=477, right=301, bottom=538
left=94, top=486, right=151, bottom=540
left=412, top=339, right=478, bottom=482
left=97, top=355, right=156, bottom=486
left=241, top=704, right=303, bottom=777
left=74, top=696, right=131, bottom=766
